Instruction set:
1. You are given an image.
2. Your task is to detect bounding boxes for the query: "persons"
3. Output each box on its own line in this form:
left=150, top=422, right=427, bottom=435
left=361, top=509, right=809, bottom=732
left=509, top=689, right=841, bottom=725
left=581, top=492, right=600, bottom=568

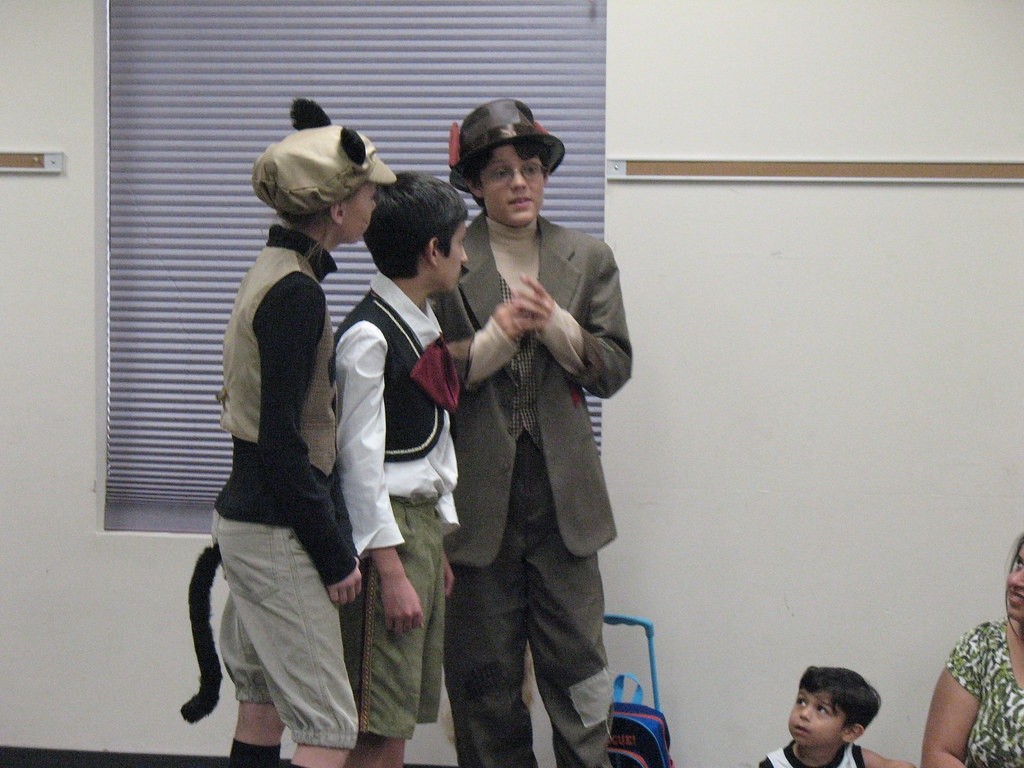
left=432, top=97, right=633, bottom=768
left=338, top=169, right=468, bottom=768
left=921, top=535, right=1024, bottom=768
left=757, top=666, right=915, bottom=768
left=178, top=96, right=397, bottom=768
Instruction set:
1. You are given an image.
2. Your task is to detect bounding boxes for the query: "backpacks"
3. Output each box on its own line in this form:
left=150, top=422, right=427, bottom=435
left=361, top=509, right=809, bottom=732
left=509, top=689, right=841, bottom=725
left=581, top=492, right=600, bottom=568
left=601, top=672, right=674, bottom=768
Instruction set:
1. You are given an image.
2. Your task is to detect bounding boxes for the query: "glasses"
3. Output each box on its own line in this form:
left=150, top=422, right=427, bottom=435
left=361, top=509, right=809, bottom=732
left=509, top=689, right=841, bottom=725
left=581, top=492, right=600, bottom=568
left=479, top=164, right=547, bottom=181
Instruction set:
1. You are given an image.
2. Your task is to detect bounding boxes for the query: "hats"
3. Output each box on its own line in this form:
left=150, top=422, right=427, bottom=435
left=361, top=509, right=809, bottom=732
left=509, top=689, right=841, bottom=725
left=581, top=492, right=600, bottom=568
left=447, top=99, right=566, bottom=194
left=250, top=98, right=398, bottom=214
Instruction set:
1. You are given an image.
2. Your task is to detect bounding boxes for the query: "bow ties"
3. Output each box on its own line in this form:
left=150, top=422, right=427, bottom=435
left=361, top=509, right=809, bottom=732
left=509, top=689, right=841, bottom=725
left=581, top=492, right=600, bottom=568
left=409, top=331, right=460, bottom=412
